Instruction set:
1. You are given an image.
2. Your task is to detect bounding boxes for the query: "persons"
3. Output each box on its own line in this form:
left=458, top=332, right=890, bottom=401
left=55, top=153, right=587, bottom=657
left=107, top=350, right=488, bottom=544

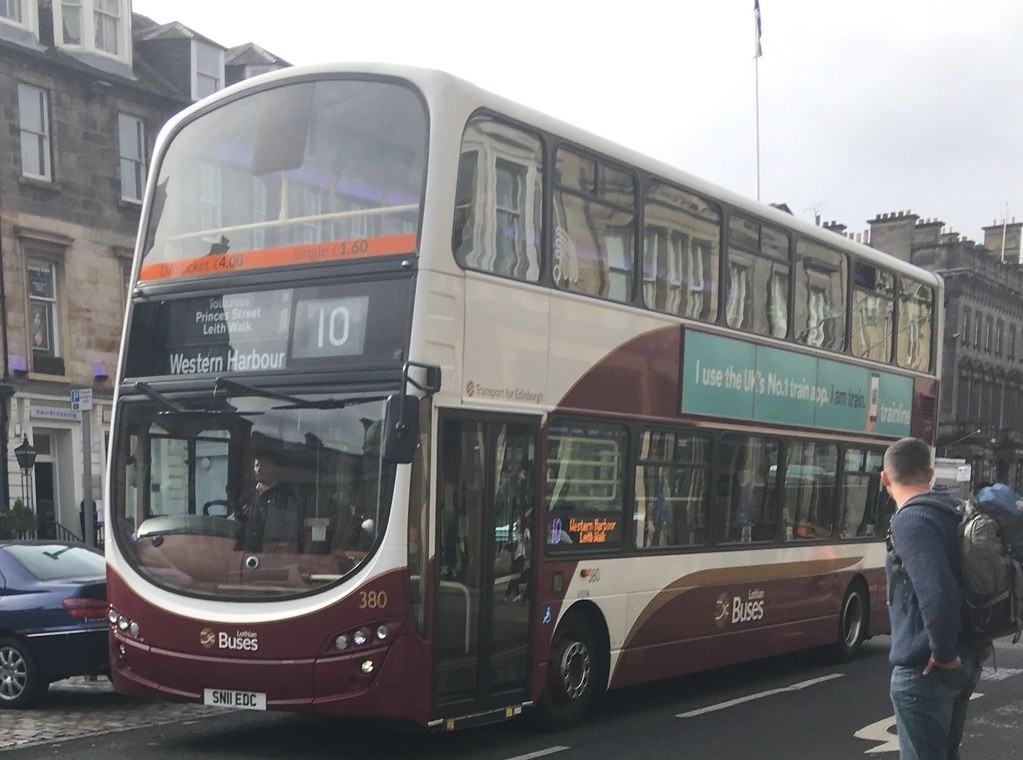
left=880, top=438, right=990, bottom=760
left=232, top=453, right=297, bottom=552
left=503, top=513, right=531, bottom=603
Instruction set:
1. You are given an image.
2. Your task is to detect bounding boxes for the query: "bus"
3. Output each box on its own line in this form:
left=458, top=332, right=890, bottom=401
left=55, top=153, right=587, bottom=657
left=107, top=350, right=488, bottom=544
left=104, top=61, right=951, bottom=734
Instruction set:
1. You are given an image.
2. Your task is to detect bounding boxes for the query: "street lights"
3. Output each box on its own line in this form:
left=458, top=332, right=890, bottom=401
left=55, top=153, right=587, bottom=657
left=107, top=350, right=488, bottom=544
left=14, top=433, right=38, bottom=542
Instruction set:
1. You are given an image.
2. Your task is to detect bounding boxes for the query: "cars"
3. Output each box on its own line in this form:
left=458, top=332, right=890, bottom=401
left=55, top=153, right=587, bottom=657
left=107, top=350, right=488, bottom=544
left=0, top=540, right=110, bottom=710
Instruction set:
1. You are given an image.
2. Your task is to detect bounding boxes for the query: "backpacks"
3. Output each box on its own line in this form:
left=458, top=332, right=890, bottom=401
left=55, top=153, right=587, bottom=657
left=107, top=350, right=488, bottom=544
left=886, top=483, right=1022, bottom=640
